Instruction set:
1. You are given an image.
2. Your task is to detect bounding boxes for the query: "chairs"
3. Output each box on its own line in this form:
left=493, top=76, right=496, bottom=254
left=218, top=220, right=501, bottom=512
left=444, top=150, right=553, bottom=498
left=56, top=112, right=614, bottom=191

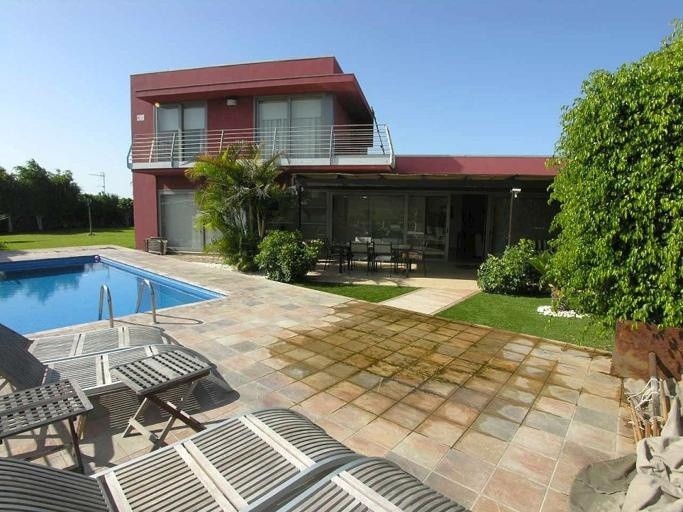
left=0, top=324, right=233, bottom=428
left=322, top=232, right=428, bottom=278
